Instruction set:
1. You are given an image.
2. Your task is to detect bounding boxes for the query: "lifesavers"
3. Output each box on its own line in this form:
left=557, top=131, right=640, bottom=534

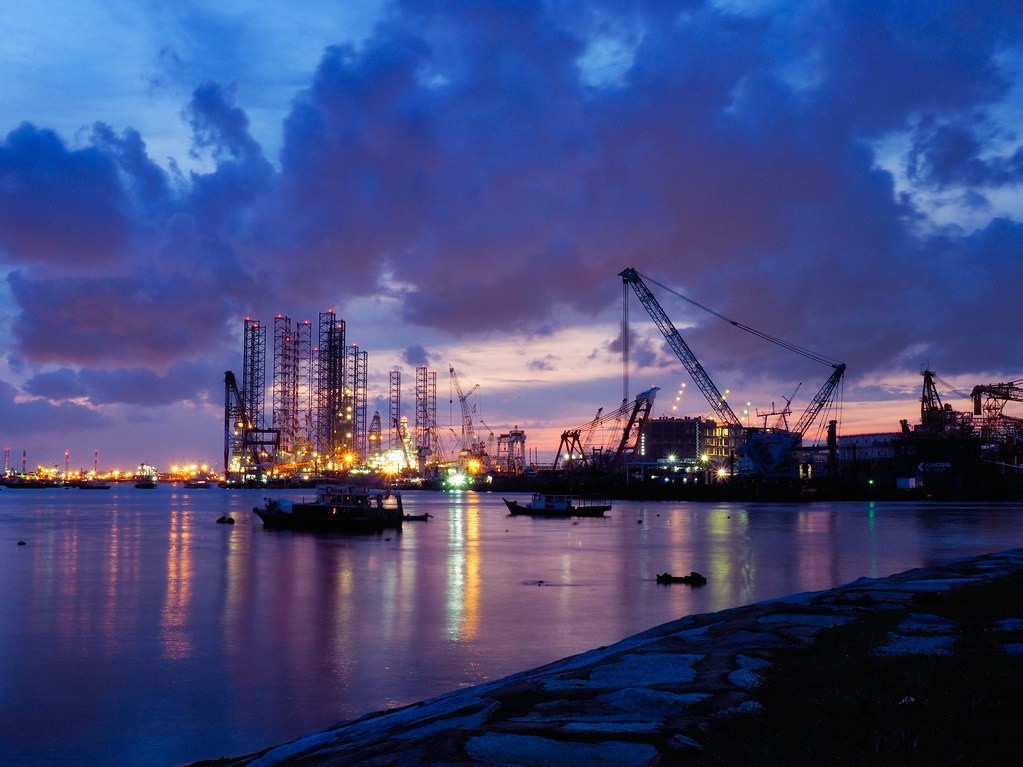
left=326, top=486, right=333, bottom=493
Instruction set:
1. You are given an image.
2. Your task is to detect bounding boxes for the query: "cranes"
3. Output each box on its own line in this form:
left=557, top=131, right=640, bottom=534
left=554, top=266, right=848, bottom=478
left=447, top=361, right=495, bottom=479
left=915, top=360, right=1023, bottom=444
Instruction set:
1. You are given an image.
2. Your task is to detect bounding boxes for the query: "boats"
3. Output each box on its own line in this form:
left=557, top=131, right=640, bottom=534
left=499, top=491, right=613, bottom=519
left=183, top=475, right=212, bottom=489
left=135, top=480, right=157, bottom=490
left=3, top=477, right=45, bottom=489
left=79, top=480, right=111, bottom=490
left=252, top=483, right=403, bottom=534
